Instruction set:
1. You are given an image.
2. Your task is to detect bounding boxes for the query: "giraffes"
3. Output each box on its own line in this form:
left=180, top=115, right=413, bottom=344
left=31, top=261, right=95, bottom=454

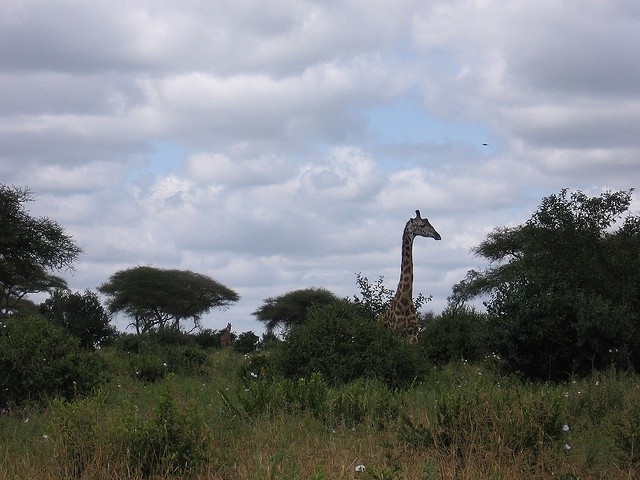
left=221, top=322, right=232, bottom=349
left=376, top=210, right=442, bottom=347
